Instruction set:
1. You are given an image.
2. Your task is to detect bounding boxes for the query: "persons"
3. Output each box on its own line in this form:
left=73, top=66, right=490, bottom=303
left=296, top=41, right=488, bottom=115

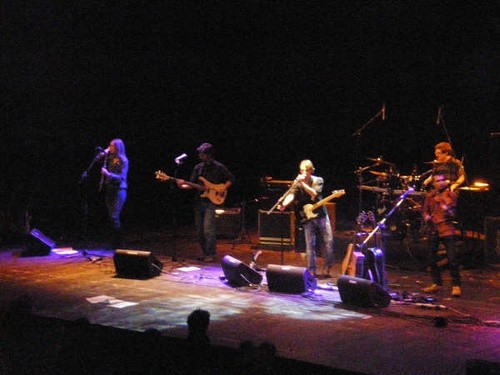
left=277, top=159, right=334, bottom=277
left=421, top=169, right=461, bottom=297
left=100, top=138, right=131, bottom=250
left=422, top=141, right=466, bottom=192
left=175, top=143, right=235, bottom=262
left=0, top=308, right=277, bottom=375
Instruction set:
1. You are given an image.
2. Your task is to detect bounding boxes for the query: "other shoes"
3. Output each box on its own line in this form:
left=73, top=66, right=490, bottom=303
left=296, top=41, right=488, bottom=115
left=310, top=268, right=315, bottom=276
left=196, top=254, right=206, bottom=260
left=451, top=286, right=461, bottom=296
left=111, top=237, right=122, bottom=247
left=322, top=266, right=330, bottom=275
left=423, top=284, right=441, bottom=292
left=206, top=255, right=216, bottom=262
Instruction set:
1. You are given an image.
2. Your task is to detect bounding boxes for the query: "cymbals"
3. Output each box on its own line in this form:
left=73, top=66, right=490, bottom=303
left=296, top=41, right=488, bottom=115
left=401, top=175, right=421, bottom=180
left=423, top=162, right=445, bottom=165
left=368, top=170, right=389, bottom=176
left=367, top=157, right=394, bottom=166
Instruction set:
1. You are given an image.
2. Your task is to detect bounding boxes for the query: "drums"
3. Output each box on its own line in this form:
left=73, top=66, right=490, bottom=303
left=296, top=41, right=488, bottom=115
left=378, top=182, right=392, bottom=202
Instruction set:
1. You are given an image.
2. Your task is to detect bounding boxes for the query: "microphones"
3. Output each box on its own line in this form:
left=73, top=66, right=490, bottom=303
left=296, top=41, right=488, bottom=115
left=436, top=105, right=442, bottom=124
left=175, top=153, right=187, bottom=161
left=381, top=103, right=386, bottom=121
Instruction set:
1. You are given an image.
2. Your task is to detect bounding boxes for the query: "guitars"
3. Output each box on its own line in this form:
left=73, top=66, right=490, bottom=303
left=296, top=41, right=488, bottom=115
left=99, top=152, right=110, bottom=191
left=296, top=189, right=346, bottom=226
left=426, top=178, right=465, bottom=233
left=154, top=172, right=229, bottom=206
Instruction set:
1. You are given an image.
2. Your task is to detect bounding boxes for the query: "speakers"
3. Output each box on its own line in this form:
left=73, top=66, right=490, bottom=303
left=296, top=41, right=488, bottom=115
left=338, top=275, right=392, bottom=307
left=266, top=263, right=317, bottom=292
left=484, top=216, right=500, bottom=266
left=29, top=228, right=56, bottom=255
left=114, top=249, right=162, bottom=278
left=222, top=255, right=262, bottom=285
